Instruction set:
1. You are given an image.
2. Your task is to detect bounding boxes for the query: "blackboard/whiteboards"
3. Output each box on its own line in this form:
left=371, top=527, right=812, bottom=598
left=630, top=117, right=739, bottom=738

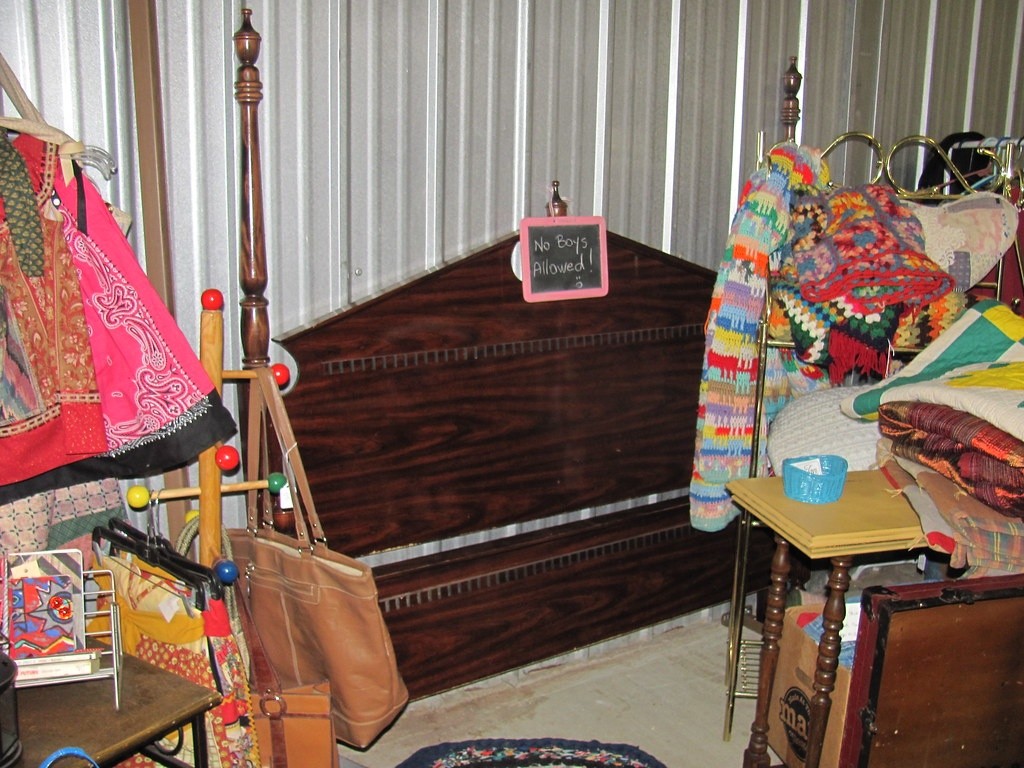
left=520, top=217, right=609, bottom=303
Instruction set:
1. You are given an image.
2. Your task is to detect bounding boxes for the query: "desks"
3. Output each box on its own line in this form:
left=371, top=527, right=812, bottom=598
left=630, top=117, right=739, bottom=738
left=18, top=638, right=226, bottom=768
left=725, top=470, right=929, bottom=768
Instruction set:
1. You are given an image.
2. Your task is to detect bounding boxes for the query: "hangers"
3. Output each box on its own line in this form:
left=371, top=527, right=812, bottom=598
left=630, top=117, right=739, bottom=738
left=936, top=136, right=1024, bottom=209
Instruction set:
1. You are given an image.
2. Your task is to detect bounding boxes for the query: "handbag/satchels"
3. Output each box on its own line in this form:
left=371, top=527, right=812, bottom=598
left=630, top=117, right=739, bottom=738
left=213, top=559, right=340, bottom=768
left=225, top=366, right=410, bottom=751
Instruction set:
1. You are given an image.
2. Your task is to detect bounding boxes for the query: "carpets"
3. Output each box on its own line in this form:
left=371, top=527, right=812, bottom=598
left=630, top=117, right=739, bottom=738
left=393, top=737, right=666, bottom=768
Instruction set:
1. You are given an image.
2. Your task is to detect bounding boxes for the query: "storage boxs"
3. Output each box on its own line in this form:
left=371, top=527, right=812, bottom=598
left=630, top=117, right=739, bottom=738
left=764, top=601, right=852, bottom=768
